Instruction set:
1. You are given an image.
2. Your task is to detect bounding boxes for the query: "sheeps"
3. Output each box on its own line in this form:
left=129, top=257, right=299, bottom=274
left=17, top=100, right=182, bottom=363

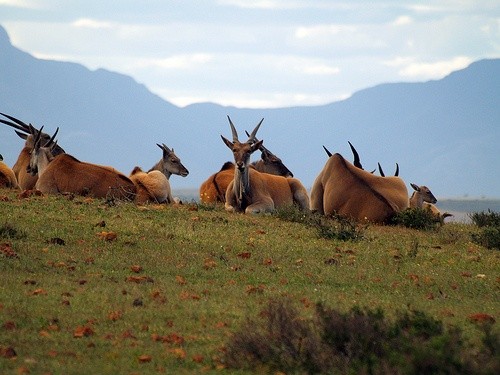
left=221, top=114, right=311, bottom=217
left=130, top=142, right=190, bottom=205
left=199, top=130, right=293, bottom=209
left=311, top=141, right=454, bottom=226
left=0, top=113, right=137, bottom=202
